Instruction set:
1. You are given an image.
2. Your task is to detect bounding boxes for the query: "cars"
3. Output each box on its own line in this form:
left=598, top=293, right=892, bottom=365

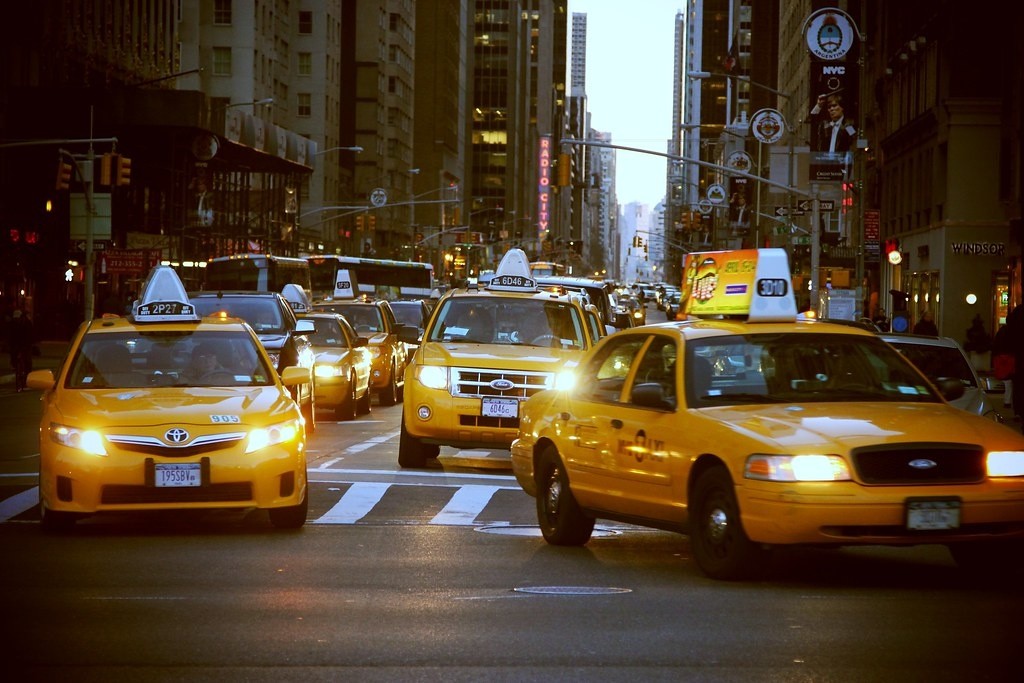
left=382, top=297, right=435, bottom=360
left=480, top=265, right=687, bottom=337
left=512, top=249, right=1024, bottom=581
left=181, top=289, right=321, bottom=432
left=24, top=262, right=314, bottom=531
left=322, top=267, right=419, bottom=407
left=872, top=332, right=998, bottom=426
left=397, top=244, right=594, bottom=472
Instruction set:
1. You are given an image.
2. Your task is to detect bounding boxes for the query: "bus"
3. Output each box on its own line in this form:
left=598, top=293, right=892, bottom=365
left=280, top=284, right=375, bottom=420
left=203, top=254, right=316, bottom=303
left=307, top=251, right=439, bottom=303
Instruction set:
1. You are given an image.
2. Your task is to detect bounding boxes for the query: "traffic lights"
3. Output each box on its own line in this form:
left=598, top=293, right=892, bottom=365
left=591, top=172, right=600, bottom=189
left=638, top=236, right=644, bottom=249
left=644, top=244, right=648, bottom=253
left=882, top=239, right=900, bottom=256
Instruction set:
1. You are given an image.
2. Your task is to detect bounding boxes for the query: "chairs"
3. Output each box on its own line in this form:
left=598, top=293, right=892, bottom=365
left=80, top=345, right=150, bottom=390
left=670, top=356, right=711, bottom=405
left=454, top=308, right=493, bottom=345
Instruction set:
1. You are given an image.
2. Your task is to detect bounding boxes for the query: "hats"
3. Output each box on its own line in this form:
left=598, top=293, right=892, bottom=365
left=13, top=309, right=22, bottom=318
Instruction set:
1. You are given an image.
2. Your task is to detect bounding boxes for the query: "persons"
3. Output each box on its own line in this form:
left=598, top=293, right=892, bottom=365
left=913, top=312, right=939, bottom=336
left=874, top=309, right=887, bottom=332
left=544, top=310, right=569, bottom=348
left=176, top=346, right=234, bottom=386
left=9, top=311, right=34, bottom=391
left=991, top=303, right=1024, bottom=420
left=98, top=346, right=150, bottom=386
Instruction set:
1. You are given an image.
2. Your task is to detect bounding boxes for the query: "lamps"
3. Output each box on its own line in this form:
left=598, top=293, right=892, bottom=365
left=314, top=146, right=364, bottom=156
left=207, top=97, right=274, bottom=112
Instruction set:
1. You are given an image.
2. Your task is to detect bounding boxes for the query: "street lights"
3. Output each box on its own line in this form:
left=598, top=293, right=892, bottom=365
left=686, top=69, right=799, bottom=281
left=373, top=168, right=535, bottom=263
left=681, top=122, right=765, bottom=251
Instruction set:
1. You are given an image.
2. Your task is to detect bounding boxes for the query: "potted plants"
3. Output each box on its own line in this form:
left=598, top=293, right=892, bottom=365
left=963, top=313, right=991, bottom=372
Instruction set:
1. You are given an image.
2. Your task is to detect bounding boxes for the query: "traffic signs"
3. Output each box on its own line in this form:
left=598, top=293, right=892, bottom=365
left=796, top=199, right=839, bottom=214
left=645, top=255, right=648, bottom=261
left=775, top=206, right=807, bottom=216
